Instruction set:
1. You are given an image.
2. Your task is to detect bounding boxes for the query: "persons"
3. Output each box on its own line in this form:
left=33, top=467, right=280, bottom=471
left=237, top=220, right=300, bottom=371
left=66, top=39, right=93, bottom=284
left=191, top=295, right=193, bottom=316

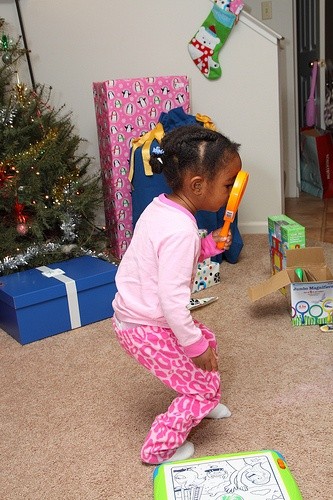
left=110, top=124, right=243, bottom=465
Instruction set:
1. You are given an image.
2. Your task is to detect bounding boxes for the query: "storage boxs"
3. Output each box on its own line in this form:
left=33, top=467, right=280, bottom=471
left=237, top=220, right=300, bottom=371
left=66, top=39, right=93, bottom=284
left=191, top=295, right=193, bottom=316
left=268, top=214, right=305, bottom=298
left=92, top=76, right=190, bottom=261
left=247, top=246, right=333, bottom=326
left=192, top=262, right=221, bottom=294
left=0, top=255, right=118, bottom=346
left=198, top=229, right=210, bottom=269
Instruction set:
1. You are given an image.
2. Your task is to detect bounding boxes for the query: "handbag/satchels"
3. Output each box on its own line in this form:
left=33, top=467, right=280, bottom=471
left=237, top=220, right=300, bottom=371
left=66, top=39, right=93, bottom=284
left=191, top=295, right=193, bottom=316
left=300, top=125, right=333, bottom=197
